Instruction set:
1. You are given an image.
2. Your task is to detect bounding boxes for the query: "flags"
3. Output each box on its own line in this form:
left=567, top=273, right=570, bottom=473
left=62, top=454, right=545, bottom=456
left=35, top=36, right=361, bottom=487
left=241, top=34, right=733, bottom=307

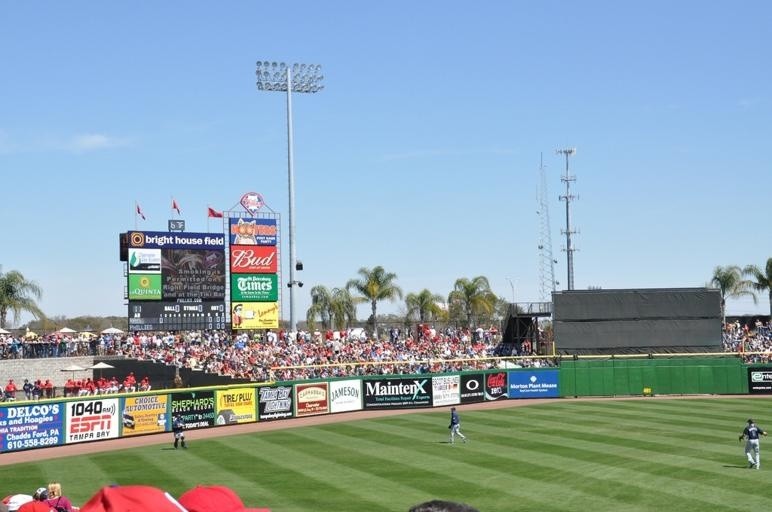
left=172, top=200, right=180, bottom=215
left=136, top=203, right=146, bottom=220
left=208, top=207, right=222, bottom=217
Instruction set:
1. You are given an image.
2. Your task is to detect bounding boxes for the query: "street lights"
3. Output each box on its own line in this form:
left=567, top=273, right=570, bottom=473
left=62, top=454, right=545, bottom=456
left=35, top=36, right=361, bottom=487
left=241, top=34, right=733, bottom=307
left=255, top=60, right=323, bottom=341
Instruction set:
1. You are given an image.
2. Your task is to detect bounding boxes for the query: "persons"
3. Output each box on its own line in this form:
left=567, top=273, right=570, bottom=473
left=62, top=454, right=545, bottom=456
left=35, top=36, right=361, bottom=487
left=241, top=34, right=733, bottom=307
left=738, top=417, right=768, bottom=470
left=46, top=482, right=73, bottom=512
left=233, top=304, right=242, bottom=328
left=33, top=487, right=59, bottom=512
left=173, top=414, right=188, bottom=449
left=721, top=318, right=772, bottom=364
left=409, top=498, right=481, bottom=510
left=448, top=407, right=466, bottom=446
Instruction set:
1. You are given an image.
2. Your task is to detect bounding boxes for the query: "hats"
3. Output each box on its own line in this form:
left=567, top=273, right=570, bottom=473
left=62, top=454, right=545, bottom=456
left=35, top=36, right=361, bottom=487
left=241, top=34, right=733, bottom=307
left=78, top=484, right=188, bottom=512
left=2, top=494, right=37, bottom=511
left=34, top=487, right=48, bottom=499
left=177, top=484, right=271, bottom=512
left=16, top=500, right=59, bottom=512
